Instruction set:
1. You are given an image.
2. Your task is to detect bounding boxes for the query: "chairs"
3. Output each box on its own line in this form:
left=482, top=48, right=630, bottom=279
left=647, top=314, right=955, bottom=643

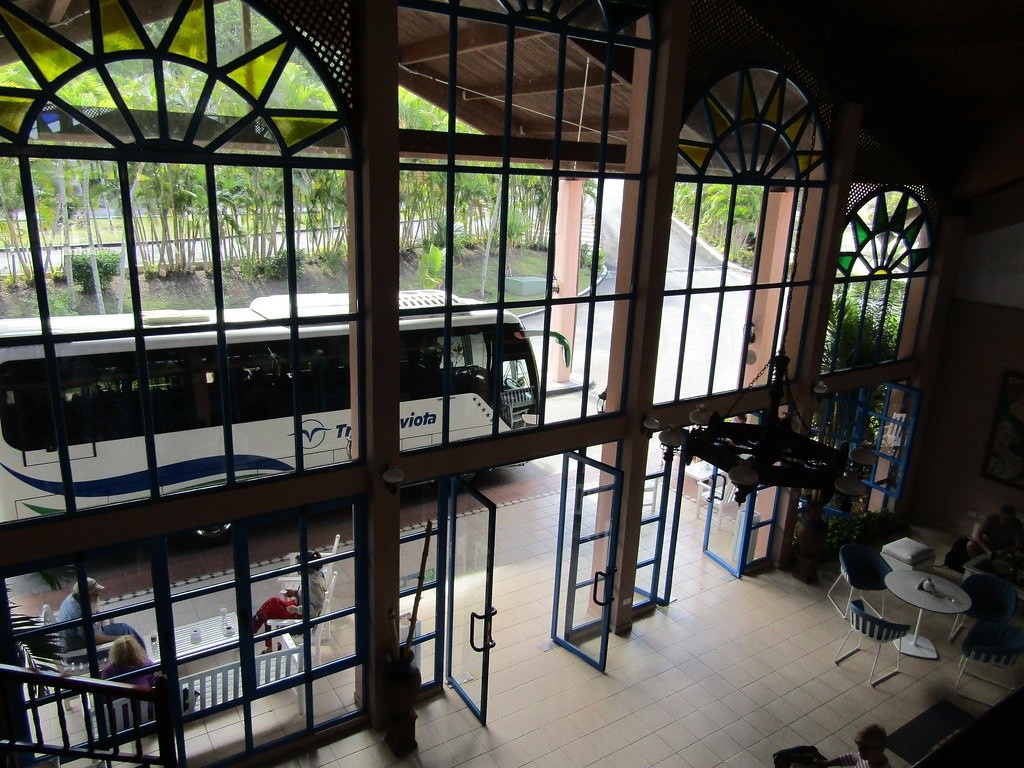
left=827, top=542, right=894, bottom=619
left=833, top=598, right=912, bottom=687
left=42, top=605, right=117, bottom=712
left=267, top=571, right=338, bottom=667
left=278, top=534, right=340, bottom=592
left=954, top=619, right=1024, bottom=707
left=948, top=573, right=1018, bottom=644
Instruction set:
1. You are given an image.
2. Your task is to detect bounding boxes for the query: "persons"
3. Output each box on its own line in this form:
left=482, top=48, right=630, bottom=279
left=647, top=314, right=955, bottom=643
left=966, top=504, right=1024, bottom=561
left=101, top=636, right=158, bottom=721
left=813, top=724, right=892, bottom=768
left=252, top=550, right=327, bottom=655
left=57, top=577, right=148, bottom=665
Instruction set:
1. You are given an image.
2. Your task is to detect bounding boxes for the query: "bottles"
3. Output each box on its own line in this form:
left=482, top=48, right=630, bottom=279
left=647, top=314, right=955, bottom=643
left=151, top=634, right=158, bottom=652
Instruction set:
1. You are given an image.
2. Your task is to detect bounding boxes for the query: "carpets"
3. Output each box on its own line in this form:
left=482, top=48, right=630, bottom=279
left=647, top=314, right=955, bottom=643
left=885, top=700, right=975, bottom=766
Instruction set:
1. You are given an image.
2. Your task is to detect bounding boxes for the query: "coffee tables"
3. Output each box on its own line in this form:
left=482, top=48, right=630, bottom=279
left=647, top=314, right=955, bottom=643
left=964, top=553, right=1024, bottom=600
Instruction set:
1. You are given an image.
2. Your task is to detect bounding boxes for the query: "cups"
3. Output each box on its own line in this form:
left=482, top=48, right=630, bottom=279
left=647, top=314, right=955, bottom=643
left=225, top=626, right=232, bottom=633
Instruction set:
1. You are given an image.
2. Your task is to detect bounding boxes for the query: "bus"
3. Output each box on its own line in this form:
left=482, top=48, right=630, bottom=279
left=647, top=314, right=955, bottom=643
left=0, top=288, right=572, bottom=548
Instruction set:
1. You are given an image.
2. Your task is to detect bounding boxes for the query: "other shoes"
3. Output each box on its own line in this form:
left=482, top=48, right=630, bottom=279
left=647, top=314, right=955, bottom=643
left=260, top=642, right=283, bottom=653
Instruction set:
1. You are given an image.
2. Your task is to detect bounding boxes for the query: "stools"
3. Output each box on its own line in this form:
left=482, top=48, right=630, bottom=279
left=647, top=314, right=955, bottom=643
left=880, top=537, right=935, bottom=574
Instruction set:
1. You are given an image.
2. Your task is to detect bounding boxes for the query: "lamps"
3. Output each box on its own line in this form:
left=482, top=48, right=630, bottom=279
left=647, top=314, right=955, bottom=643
left=810, top=379, right=828, bottom=403
left=382, top=465, right=404, bottom=498
left=658, top=108, right=876, bottom=515
left=641, top=413, right=660, bottom=442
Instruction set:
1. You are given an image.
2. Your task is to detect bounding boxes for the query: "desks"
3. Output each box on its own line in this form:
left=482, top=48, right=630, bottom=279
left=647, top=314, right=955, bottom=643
left=149, top=612, right=239, bottom=663
left=884, top=569, right=972, bottom=660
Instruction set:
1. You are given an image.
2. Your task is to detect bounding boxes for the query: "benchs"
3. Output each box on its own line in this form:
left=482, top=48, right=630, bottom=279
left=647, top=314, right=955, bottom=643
left=79, top=632, right=305, bottom=739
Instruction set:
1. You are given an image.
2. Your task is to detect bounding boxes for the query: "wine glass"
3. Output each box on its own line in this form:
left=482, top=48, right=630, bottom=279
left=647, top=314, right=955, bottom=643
left=220, top=607, right=227, bottom=624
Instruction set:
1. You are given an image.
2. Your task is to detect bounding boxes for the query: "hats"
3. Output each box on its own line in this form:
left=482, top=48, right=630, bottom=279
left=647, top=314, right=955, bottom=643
left=72, top=577, right=104, bottom=594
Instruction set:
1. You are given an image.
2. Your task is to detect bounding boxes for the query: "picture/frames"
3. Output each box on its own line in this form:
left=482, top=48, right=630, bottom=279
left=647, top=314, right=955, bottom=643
left=980, top=369, right=1024, bottom=493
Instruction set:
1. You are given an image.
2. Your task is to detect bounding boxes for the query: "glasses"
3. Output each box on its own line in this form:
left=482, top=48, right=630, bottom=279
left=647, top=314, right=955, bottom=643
left=854, top=737, right=884, bottom=752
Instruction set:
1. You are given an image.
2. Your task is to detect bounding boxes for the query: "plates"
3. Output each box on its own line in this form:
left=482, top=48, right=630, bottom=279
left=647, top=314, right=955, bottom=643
left=223, top=628, right=235, bottom=637
